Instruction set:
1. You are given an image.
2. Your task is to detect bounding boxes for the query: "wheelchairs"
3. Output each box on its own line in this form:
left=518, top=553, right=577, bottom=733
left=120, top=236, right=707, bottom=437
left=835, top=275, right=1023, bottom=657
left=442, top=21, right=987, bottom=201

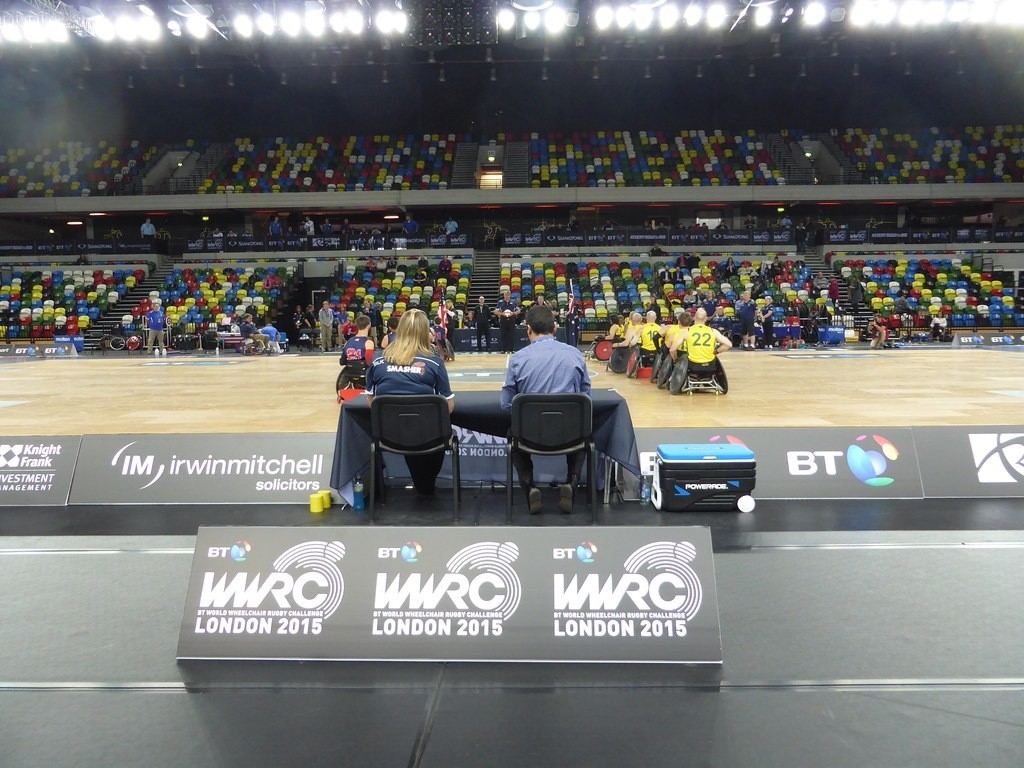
left=431, top=337, right=455, bottom=362
left=583, top=334, right=688, bottom=389
left=244, top=333, right=271, bottom=356
left=669, top=351, right=729, bottom=395
left=335, top=355, right=374, bottom=401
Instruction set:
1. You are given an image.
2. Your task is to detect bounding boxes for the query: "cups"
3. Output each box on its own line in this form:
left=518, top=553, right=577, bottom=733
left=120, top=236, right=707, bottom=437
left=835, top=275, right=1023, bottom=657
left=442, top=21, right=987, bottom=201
left=309, top=494, right=323, bottom=513
left=317, top=490, right=331, bottom=508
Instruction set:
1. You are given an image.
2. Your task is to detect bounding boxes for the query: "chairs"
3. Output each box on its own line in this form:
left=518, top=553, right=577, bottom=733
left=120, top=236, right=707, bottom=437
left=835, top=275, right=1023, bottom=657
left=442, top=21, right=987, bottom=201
left=506, top=392, right=598, bottom=524
left=368, top=391, right=461, bottom=520
left=13, top=126, right=1023, bottom=337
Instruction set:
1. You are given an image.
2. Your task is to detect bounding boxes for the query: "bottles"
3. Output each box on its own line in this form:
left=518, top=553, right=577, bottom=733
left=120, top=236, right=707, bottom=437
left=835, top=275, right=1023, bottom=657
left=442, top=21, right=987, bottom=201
left=353, top=474, right=365, bottom=512
left=641, top=475, right=650, bottom=504
left=215, top=347, right=219, bottom=355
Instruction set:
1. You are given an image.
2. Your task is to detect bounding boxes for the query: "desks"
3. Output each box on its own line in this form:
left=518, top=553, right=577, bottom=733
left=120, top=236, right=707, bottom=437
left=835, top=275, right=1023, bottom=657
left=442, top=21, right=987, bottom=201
left=329, top=390, right=642, bottom=503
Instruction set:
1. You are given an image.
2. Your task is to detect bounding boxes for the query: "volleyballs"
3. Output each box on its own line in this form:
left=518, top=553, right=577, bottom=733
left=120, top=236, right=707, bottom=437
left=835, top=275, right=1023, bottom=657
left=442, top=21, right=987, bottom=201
left=504, top=309, right=511, bottom=317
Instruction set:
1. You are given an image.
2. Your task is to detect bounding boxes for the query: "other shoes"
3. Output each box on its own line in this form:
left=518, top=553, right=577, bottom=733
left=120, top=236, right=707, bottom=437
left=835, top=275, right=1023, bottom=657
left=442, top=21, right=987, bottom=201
left=147, top=350, right=152, bottom=354
left=742, top=345, right=755, bottom=351
left=558, top=483, right=574, bottom=513
left=526, top=486, right=543, bottom=514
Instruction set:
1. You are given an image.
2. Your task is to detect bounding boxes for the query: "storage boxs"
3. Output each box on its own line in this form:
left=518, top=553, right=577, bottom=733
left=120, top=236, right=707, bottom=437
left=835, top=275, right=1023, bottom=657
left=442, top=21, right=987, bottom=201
left=650, top=443, right=756, bottom=512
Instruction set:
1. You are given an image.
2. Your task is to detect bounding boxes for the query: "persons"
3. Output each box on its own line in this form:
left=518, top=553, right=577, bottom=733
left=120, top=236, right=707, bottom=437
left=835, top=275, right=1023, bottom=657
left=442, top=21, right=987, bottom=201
left=364, top=308, right=454, bottom=505
left=498, top=305, right=591, bottom=515
left=141, top=215, right=946, bottom=396
